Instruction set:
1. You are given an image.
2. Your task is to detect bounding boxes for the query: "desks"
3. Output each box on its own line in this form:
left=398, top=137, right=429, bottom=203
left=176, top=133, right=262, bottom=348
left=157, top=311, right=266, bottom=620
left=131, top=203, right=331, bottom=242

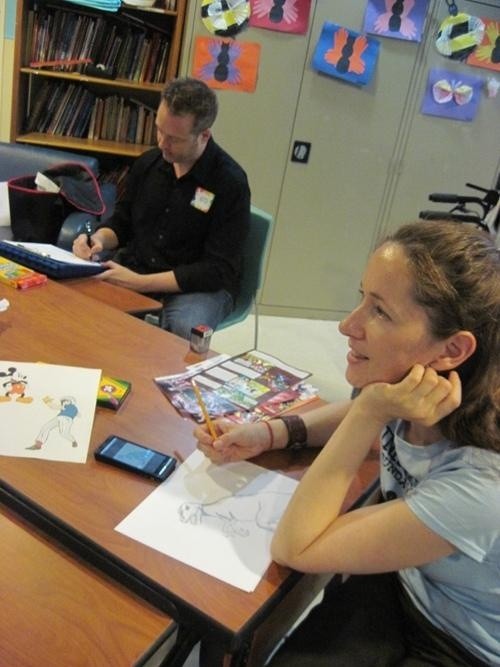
left=0, top=253, right=389, bottom=667
left=0, top=503, right=181, bottom=667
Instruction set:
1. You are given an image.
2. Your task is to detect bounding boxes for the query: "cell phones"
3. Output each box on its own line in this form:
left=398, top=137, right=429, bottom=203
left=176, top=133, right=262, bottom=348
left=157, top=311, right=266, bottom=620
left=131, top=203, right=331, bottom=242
left=94, top=434, right=177, bottom=481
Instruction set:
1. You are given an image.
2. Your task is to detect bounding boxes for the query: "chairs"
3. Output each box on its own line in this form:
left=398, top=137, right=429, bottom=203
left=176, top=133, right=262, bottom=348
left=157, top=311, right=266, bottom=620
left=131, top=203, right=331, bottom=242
left=144, top=204, right=274, bottom=349
left=0, top=141, right=99, bottom=243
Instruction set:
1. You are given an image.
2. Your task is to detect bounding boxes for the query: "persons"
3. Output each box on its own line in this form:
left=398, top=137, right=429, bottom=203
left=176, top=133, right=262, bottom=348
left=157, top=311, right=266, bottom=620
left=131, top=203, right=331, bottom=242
left=74, top=79, right=251, bottom=339
left=192, top=221, right=499, bottom=667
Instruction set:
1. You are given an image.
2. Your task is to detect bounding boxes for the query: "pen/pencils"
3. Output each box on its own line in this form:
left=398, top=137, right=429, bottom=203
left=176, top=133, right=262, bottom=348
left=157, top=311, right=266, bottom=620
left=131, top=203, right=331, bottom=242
left=190, top=379, right=218, bottom=440
left=85, top=220, right=92, bottom=262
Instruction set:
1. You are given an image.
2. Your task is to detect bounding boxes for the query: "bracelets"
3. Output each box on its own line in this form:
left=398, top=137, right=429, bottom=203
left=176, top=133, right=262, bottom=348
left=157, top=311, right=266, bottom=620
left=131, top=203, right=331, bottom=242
left=273, top=413, right=309, bottom=454
left=256, top=420, right=274, bottom=454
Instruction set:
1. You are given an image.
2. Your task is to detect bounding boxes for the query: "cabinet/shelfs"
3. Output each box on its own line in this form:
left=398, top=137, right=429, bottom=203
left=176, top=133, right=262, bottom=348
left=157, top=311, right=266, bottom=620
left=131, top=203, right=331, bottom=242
left=6, top=0, right=200, bottom=193
left=178, top=0, right=500, bottom=321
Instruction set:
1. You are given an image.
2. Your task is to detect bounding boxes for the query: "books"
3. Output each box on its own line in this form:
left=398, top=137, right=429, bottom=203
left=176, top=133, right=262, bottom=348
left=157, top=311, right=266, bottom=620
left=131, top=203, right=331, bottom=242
left=0, top=241, right=107, bottom=281
left=23, top=1, right=177, bottom=149
